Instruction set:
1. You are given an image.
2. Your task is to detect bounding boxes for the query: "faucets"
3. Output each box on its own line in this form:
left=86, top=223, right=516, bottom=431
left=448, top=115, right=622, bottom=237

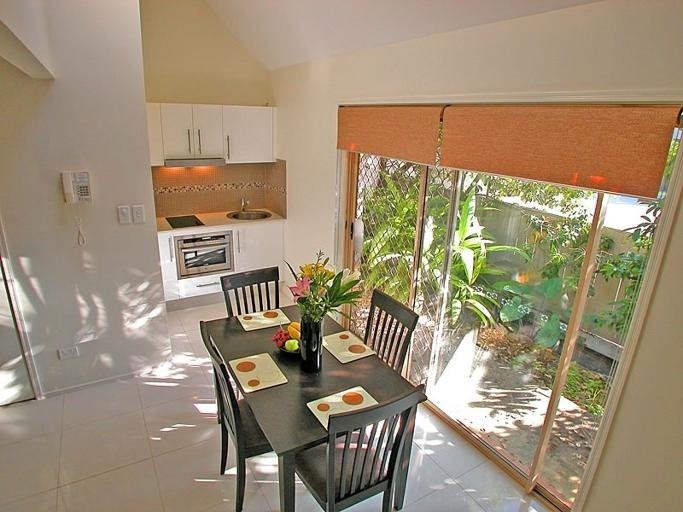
left=241, top=194, right=246, bottom=212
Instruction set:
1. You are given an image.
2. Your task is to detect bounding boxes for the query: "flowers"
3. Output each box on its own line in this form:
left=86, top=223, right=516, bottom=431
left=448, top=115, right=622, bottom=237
left=282, top=249, right=367, bottom=326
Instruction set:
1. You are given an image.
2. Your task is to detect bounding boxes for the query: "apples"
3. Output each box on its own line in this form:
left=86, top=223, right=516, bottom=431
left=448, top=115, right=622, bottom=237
left=285, top=339, right=299, bottom=351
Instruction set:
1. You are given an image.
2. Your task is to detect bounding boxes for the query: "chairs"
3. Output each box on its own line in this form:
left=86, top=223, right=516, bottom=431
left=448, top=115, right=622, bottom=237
left=220, top=267, right=279, bottom=319
left=295, top=386, right=426, bottom=512
left=362, top=289, right=421, bottom=374
left=199, top=320, right=274, bottom=510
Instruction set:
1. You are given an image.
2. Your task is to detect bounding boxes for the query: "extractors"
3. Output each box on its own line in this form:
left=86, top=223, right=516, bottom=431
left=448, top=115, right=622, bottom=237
left=163, top=158, right=226, bottom=168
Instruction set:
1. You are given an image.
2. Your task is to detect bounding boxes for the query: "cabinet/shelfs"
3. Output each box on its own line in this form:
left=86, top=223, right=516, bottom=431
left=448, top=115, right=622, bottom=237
left=224, top=105, right=279, bottom=163
left=145, top=103, right=224, bottom=165
left=158, top=226, right=236, bottom=300
left=231, top=221, right=285, bottom=294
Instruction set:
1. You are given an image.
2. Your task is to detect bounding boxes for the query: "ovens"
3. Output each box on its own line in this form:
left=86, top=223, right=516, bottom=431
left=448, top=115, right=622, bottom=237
left=173, top=230, right=235, bottom=279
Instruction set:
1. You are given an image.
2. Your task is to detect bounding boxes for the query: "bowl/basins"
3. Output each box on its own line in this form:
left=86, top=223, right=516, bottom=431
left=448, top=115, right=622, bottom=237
left=280, top=348, right=300, bottom=362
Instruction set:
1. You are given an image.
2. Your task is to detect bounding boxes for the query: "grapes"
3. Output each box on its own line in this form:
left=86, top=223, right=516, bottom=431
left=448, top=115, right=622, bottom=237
left=271, top=329, right=289, bottom=347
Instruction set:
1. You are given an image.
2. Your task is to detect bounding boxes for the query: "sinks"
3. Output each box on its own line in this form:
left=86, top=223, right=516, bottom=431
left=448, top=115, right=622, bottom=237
left=227, top=210, right=272, bottom=220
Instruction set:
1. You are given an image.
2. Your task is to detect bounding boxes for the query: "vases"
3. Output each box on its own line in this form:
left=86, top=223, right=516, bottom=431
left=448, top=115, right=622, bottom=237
left=300, top=319, right=323, bottom=378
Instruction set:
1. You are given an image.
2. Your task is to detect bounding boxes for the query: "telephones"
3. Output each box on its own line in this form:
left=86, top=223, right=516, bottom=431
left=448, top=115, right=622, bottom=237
left=61, top=170, right=91, bottom=203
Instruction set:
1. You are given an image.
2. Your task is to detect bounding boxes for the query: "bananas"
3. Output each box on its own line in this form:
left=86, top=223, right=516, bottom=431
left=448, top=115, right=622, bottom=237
left=288, top=322, right=300, bottom=340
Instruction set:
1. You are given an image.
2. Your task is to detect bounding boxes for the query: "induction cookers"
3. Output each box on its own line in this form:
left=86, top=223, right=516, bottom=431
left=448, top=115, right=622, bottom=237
left=164, top=215, right=204, bottom=228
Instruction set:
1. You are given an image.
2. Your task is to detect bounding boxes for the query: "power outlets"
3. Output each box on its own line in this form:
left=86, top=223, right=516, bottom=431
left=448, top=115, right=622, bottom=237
left=57, top=346, right=81, bottom=362
